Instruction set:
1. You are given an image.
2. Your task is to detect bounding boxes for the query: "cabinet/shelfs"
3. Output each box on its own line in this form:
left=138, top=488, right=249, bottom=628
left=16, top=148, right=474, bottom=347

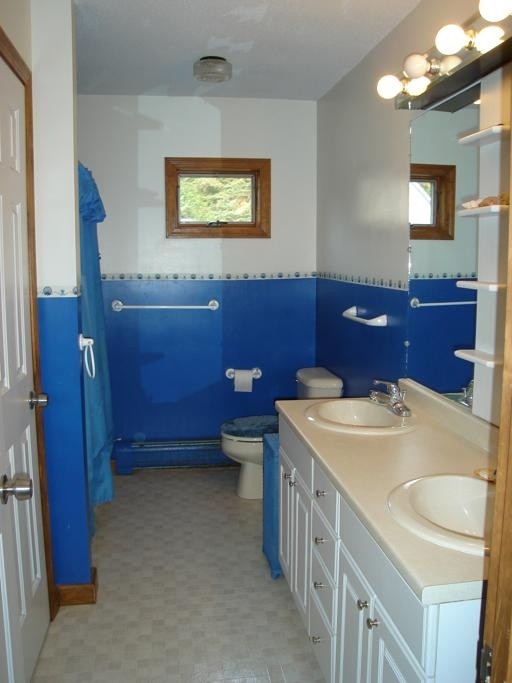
left=336, top=496, right=481, bottom=683
left=307, top=460, right=340, bottom=682
left=451, top=124, right=511, bottom=368
left=279, top=414, right=315, bottom=646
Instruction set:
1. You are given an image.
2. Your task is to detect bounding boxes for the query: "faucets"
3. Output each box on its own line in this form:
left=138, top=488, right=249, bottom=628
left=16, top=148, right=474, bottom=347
left=457, top=378, right=473, bottom=408
left=369, top=379, right=411, bottom=416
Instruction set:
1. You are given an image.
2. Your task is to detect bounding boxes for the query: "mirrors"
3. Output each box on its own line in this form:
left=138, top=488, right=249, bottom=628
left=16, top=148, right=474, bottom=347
left=402, top=58, right=512, bottom=425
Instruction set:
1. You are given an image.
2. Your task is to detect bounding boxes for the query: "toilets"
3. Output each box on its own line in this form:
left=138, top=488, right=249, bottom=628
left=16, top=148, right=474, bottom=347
left=220, top=368, right=344, bottom=499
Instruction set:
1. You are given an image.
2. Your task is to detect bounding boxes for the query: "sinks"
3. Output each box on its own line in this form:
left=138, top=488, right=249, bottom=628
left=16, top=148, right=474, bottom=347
left=386, top=472, right=497, bottom=558
left=306, top=398, right=418, bottom=437
